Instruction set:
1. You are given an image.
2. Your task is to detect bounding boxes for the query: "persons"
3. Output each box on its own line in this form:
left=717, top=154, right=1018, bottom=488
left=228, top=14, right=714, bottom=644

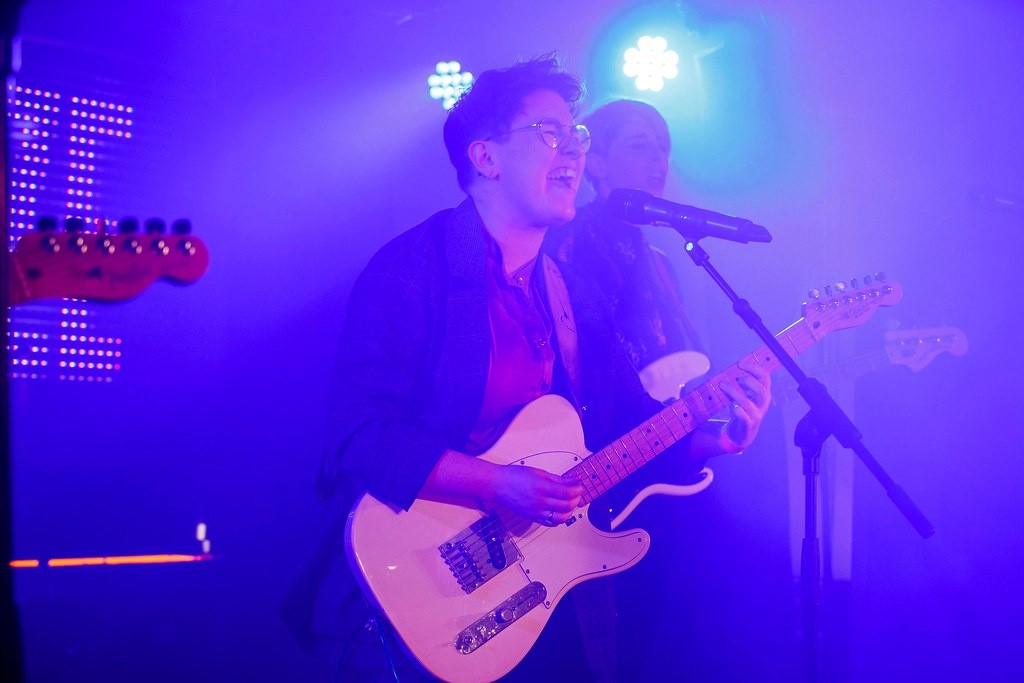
left=544, top=102, right=715, bottom=682
left=292, top=53, right=773, bottom=683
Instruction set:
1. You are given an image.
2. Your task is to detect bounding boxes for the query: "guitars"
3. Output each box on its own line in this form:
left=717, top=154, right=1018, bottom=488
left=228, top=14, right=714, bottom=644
left=345, top=268, right=902, bottom=682
left=6, top=212, right=210, bottom=307
left=708, top=325, right=970, bottom=419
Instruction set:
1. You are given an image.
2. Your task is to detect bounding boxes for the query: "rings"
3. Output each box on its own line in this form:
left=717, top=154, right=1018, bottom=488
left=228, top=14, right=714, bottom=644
left=547, top=511, right=553, bottom=524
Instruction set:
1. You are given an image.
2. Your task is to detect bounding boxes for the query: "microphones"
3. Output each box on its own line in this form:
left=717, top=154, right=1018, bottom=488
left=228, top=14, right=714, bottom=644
left=606, top=189, right=774, bottom=244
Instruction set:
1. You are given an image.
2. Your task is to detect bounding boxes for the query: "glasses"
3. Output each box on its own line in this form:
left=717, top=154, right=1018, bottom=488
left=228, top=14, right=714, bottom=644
left=485, top=119, right=592, bottom=156
